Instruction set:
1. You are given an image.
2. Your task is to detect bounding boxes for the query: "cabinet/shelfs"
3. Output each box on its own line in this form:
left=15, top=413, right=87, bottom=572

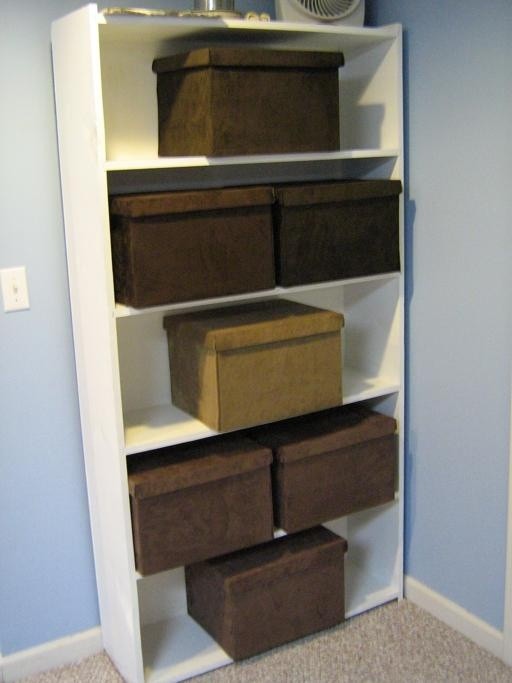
left=51, top=3, right=405, bottom=683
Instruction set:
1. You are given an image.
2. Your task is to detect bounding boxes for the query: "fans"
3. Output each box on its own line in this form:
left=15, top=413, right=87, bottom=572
left=274, top=1, right=366, bottom=28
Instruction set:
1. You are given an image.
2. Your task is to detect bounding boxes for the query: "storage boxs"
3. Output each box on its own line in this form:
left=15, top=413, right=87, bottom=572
left=239, top=407, right=396, bottom=534
left=152, top=47, right=344, bottom=157
left=185, top=525, right=348, bottom=662
left=271, top=180, right=402, bottom=288
left=126, top=431, right=274, bottom=578
left=162, top=299, right=345, bottom=433
left=108, top=186, right=276, bottom=309
